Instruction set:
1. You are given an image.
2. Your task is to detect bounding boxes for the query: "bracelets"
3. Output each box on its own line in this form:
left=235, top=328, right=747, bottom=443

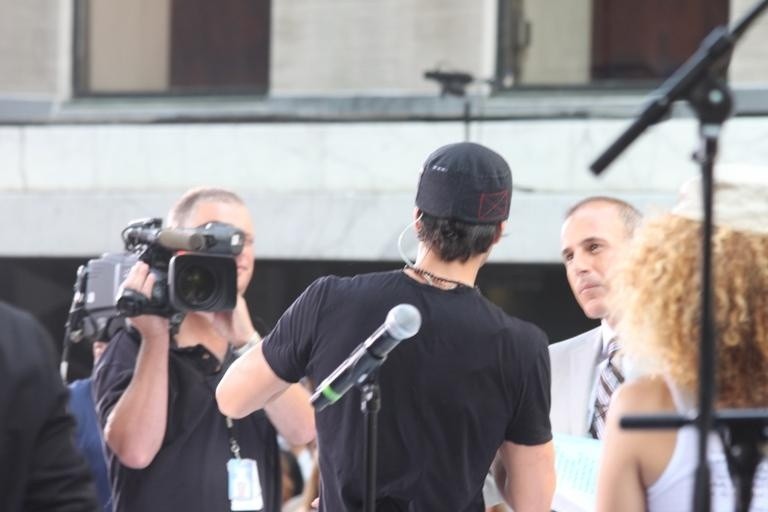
left=231, top=329, right=260, bottom=356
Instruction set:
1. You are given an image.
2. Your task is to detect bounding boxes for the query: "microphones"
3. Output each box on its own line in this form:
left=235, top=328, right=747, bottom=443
left=310, top=303, right=422, bottom=411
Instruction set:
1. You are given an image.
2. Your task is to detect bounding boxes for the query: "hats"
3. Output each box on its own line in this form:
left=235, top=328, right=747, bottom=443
left=415, top=143, right=512, bottom=226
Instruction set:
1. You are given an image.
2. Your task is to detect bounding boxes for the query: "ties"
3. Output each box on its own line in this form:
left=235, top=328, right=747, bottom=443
left=588, top=336, right=625, bottom=441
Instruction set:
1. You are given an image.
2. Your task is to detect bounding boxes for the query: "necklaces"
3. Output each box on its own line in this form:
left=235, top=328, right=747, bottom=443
left=405, top=265, right=466, bottom=288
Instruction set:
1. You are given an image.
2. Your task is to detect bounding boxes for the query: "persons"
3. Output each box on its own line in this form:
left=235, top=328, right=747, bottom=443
left=593, top=163, right=768, bottom=511
left=480, top=196, right=647, bottom=511
left=213, top=139, right=559, bottom=511
left=67, top=342, right=114, bottom=511
left=0, top=303, right=102, bottom=512
left=91, top=186, right=316, bottom=512
left=283, top=375, right=319, bottom=511
